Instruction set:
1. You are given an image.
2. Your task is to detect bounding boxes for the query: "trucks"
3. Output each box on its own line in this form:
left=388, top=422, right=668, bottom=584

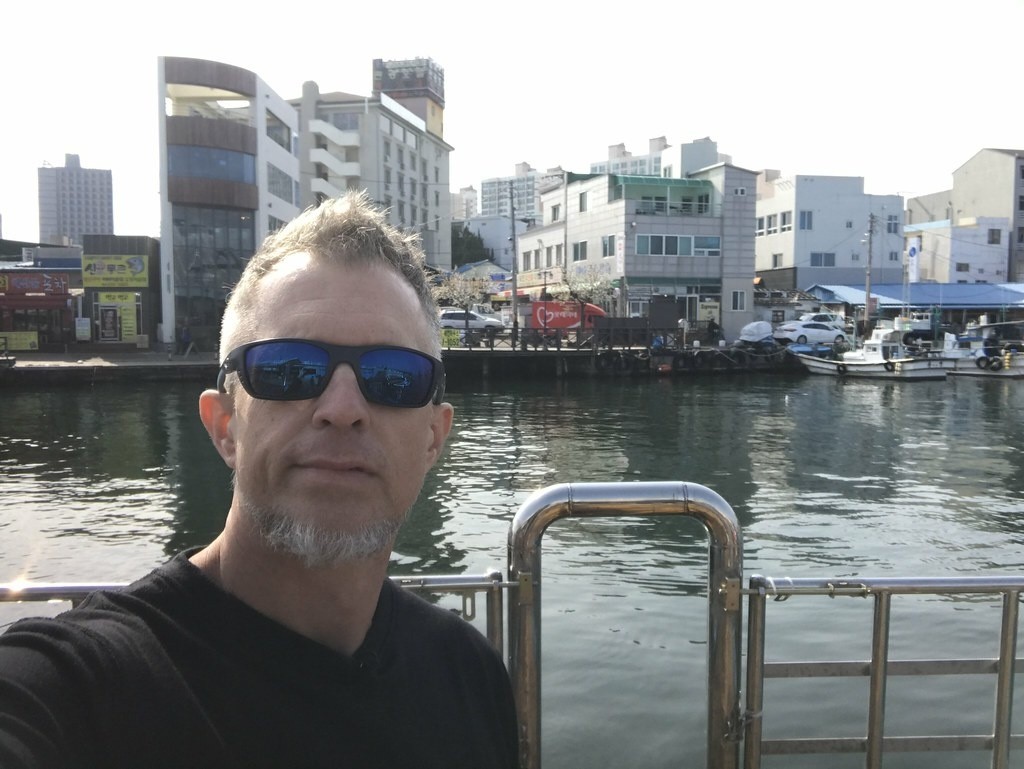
left=532, top=300, right=607, bottom=336
left=441, top=304, right=502, bottom=321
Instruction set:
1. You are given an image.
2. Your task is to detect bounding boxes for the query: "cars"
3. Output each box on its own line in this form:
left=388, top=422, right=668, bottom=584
left=779, top=313, right=845, bottom=330
left=440, top=310, right=505, bottom=334
left=844, top=316, right=893, bottom=335
left=773, top=321, right=846, bottom=345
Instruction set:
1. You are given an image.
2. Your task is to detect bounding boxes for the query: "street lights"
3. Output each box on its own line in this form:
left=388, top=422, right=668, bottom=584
left=538, top=271, right=553, bottom=350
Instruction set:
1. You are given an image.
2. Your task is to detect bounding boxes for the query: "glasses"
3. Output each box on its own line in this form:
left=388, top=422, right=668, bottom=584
left=217, top=337, right=445, bottom=408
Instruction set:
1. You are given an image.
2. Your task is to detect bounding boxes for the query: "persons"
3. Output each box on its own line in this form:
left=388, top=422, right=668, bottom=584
left=0, top=187, right=519, bottom=768
left=707, top=317, right=723, bottom=338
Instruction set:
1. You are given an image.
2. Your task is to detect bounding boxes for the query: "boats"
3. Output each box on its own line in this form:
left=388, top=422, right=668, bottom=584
left=639, top=336, right=832, bottom=374
left=797, top=312, right=958, bottom=380
left=928, top=320, right=1024, bottom=377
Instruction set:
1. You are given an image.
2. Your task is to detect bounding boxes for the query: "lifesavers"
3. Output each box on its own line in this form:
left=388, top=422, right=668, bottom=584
left=594, top=351, right=789, bottom=374
left=885, top=361, right=895, bottom=372
left=837, top=365, right=847, bottom=375
left=976, top=356, right=1003, bottom=371
left=811, top=342, right=824, bottom=356
